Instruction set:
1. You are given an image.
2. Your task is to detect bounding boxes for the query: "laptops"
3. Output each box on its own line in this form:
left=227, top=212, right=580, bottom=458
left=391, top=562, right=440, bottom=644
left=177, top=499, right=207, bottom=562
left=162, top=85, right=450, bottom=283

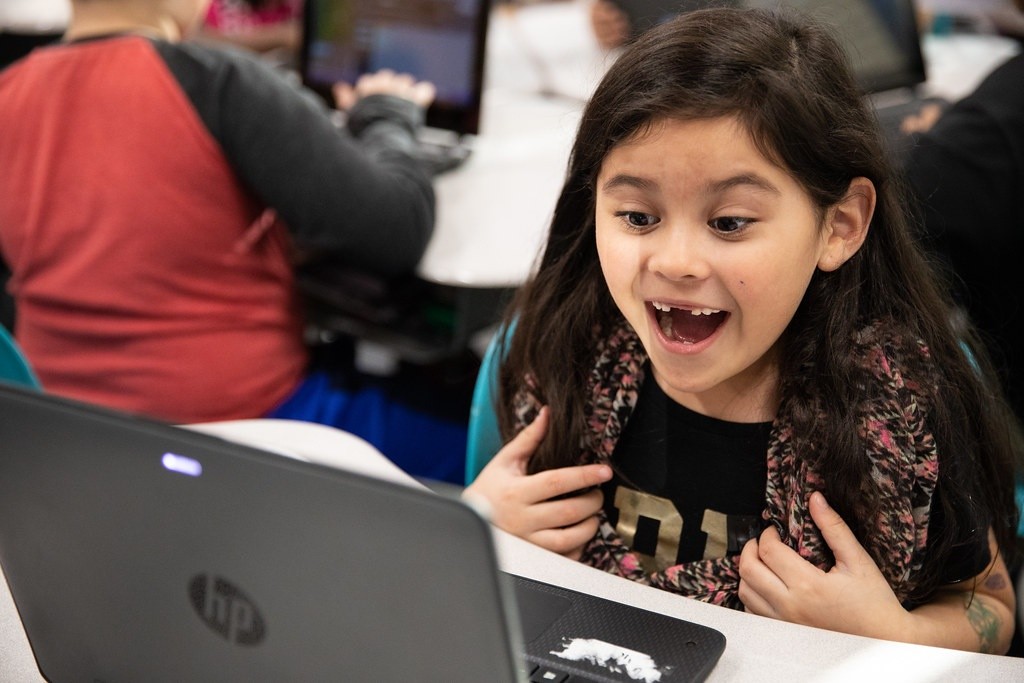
left=735, top=0, right=951, bottom=145
left=296, top=0, right=491, bottom=173
left=0, top=385, right=727, bottom=683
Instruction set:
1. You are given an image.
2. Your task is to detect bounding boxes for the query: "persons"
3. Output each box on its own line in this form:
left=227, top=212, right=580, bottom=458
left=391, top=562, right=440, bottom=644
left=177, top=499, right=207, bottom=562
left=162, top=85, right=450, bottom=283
left=4, top=0, right=468, bottom=484
left=460, top=5, right=1017, bottom=653
left=900, top=1, right=1024, bottom=441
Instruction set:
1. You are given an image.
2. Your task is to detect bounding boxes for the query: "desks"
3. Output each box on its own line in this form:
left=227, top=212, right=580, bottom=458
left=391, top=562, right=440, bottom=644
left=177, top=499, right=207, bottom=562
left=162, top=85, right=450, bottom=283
left=1, top=419, right=1024, bottom=683
left=300, top=136, right=568, bottom=358
